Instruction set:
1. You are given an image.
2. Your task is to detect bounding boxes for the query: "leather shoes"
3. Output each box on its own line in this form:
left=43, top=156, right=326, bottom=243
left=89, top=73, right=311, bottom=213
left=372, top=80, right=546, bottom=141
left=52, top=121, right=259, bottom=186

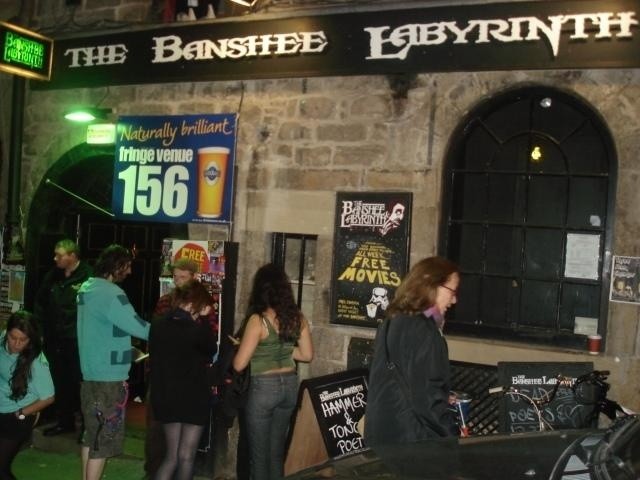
left=76, top=423, right=86, bottom=444
left=41, top=420, right=78, bottom=439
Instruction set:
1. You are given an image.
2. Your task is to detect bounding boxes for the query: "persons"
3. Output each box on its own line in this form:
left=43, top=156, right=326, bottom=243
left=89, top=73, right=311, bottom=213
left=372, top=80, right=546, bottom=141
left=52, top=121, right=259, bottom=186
left=232, top=262, right=315, bottom=480
left=144, top=279, right=217, bottom=480
left=38, top=239, right=94, bottom=443
left=0, top=308, right=55, bottom=480
left=75, top=241, right=151, bottom=480
left=361, top=255, right=462, bottom=448
left=151, top=258, right=217, bottom=368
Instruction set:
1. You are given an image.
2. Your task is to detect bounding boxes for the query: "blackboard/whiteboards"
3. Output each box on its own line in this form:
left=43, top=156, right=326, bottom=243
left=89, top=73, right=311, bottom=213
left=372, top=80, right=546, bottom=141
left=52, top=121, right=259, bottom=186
left=497, top=361, right=593, bottom=438
left=308, top=370, right=368, bottom=458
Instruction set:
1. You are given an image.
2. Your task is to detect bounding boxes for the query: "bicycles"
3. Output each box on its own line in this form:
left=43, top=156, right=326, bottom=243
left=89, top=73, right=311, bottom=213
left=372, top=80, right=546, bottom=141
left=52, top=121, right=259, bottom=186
left=443, top=371, right=574, bottom=437
left=566, top=364, right=638, bottom=427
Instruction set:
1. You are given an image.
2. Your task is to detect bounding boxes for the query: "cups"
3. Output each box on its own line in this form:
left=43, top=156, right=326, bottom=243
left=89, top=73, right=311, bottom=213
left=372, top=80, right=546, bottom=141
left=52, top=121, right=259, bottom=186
left=587, top=334, right=603, bottom=355
left=195, top=146, right=231, bottom=218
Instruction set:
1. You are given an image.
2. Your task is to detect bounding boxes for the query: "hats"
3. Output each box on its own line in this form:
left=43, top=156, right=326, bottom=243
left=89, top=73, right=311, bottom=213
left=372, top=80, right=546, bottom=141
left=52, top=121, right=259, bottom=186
left=167, top=256, right=199, bottom=274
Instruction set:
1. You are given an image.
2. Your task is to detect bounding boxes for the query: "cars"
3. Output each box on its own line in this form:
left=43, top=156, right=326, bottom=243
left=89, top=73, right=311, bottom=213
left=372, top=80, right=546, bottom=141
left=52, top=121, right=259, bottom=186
left=276, top=412, right=640, bottom=480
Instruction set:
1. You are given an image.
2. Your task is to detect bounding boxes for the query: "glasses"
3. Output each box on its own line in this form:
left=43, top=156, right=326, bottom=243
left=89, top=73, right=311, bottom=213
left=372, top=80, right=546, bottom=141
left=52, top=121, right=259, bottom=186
left=437, top=283, right=458, bottom=297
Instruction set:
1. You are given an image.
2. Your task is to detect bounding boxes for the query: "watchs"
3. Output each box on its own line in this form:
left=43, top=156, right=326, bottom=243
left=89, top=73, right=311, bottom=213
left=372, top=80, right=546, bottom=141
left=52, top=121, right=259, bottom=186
left=17, top=409, right=28, bottom=423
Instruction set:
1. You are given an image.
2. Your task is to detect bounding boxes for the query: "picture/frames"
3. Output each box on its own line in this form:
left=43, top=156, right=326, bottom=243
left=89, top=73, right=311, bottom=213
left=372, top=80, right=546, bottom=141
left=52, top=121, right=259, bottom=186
left=328, top=190, right=414, bottom=329
left=608, top=255, right=640, bottom=305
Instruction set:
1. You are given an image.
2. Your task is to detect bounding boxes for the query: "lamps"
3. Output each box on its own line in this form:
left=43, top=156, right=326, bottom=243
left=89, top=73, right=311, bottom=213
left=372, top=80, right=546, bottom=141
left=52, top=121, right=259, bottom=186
left=63, top=106, right=114, bottom=124
